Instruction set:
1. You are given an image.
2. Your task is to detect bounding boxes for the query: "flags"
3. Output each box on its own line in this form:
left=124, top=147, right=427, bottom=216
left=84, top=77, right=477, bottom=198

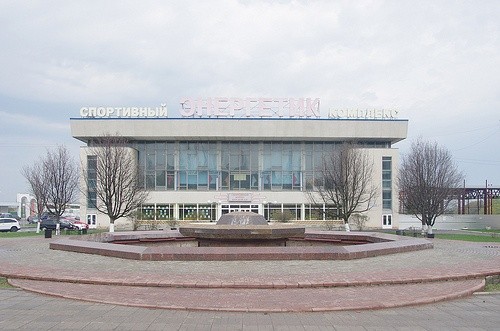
left=167, top=172, right=298, bottom=190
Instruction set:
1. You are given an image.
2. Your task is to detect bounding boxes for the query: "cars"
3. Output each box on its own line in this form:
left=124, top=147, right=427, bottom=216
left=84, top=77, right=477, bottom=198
left=1, top=213, right=22, bottom=222
left=27, top=212, right=89, bottom=231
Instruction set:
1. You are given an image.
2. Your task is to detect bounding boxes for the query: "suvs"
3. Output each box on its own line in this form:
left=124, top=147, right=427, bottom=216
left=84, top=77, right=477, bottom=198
left=0, top=218, right=21, bottom=232
